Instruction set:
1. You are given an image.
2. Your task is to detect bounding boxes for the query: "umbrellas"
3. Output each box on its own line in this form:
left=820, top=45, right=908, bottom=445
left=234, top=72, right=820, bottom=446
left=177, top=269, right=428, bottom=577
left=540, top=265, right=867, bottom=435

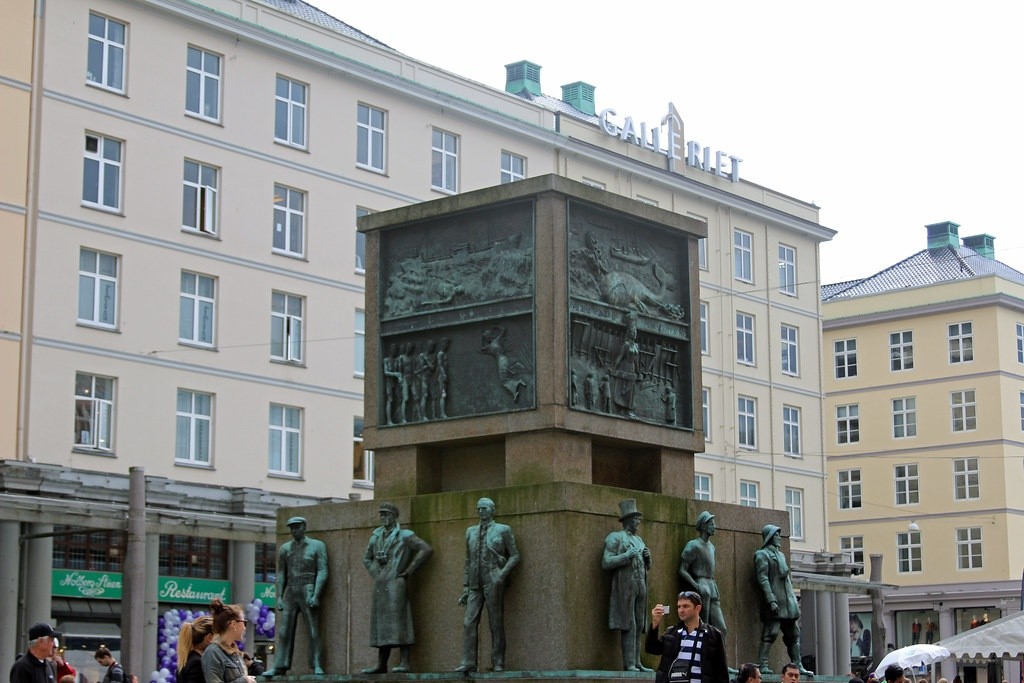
left=875, top=644, right=950, bottom=679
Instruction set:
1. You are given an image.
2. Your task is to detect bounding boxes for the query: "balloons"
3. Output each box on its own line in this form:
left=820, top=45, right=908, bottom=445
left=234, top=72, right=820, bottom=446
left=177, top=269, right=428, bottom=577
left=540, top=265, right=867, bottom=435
left=149, top=598, right=246, bottom=683
left=246, top=599, right=276, bottom=639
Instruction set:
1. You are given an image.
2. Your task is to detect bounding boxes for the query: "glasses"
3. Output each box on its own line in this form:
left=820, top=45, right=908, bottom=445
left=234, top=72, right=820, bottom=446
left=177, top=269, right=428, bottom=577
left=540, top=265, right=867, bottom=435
left=756, top=676, right=763, bottom=680
left=678, top=592, right=700, bottom=604
left=788, top=673, right=799, bottom=677
left=230, top=619, right=247, bottom=627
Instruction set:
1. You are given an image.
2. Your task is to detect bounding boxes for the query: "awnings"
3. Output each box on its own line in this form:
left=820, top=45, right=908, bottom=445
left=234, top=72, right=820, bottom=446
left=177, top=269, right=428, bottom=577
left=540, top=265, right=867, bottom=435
left=932, top=610, right=1024, bottom=664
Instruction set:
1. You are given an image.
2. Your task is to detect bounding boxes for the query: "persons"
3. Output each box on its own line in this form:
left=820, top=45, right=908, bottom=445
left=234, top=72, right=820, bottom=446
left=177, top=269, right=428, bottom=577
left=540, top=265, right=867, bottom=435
left=753, top=524, right=814, bottom=674
left=10, top=623, right=76, bottom=683
left=925, top=616, right=936, bottom=644
left=682, top=511, right=739, bottom=673
left=455, top=498, right=520, bottom=671
left=737, top=662, right=762, bottom=683
left=177, top=599, right=265, bottom=683
left=970, top=613, right=991, bottom=629
left=781, top=663, right=800, bottom=683
left=912, top=618, right=922, bottom=644
left=262, top=516, right=329, bottom=675
left=94, top=647, right=124, bottom=683
left=850, top=614, right=871, bottom=656
left=849, top=665, right=962, bottom=683
left=645, top=591, right=729, bottom=683
left=362, top=502, right=433, bottom=674
left=603, top=498, right=655, bottom=672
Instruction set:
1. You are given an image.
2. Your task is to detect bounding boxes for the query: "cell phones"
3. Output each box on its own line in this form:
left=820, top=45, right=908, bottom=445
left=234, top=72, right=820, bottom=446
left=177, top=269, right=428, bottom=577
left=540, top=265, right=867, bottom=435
left=661, top=606, right=670, bottom=614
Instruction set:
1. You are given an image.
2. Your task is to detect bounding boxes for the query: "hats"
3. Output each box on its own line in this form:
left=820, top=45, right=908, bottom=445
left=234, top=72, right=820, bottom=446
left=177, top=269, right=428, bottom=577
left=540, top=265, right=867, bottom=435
left=870, top=672, right=875, bottom=678
left=849, top=614, right=863, bottom=633
left=29, top=623, right=63, bottom=641
